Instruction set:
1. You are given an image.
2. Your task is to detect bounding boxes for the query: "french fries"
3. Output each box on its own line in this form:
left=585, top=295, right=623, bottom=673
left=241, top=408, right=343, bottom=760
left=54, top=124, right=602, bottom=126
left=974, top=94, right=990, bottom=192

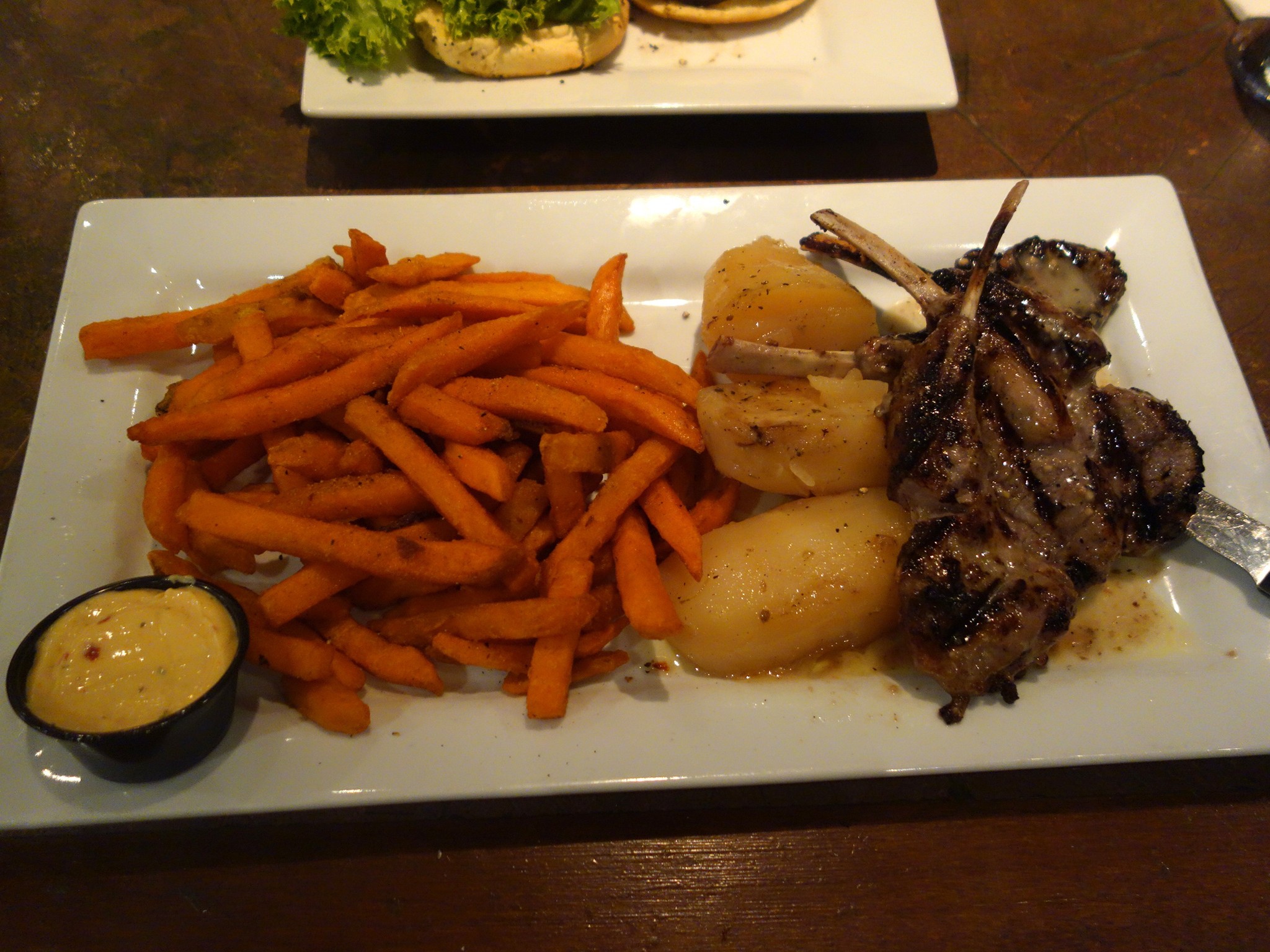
left=77, top=224, right=737, bottom=730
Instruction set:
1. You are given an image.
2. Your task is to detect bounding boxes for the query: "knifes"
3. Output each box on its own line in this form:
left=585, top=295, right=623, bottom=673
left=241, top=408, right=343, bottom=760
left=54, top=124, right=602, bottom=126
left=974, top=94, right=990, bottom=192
left=1187, top=491, right=1269, bottom=596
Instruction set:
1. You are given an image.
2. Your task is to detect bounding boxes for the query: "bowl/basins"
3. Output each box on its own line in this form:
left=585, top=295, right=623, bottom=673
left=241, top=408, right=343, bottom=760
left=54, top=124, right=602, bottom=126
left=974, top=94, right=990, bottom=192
left=5, top=576, right=252, bottom=785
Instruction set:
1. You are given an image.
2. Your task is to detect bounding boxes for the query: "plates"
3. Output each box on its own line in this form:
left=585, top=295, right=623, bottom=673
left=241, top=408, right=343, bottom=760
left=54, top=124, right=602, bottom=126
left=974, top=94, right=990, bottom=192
left=0, top=175, right=1269, bottom=832
left=299, top=0, right=957, bottom=118
